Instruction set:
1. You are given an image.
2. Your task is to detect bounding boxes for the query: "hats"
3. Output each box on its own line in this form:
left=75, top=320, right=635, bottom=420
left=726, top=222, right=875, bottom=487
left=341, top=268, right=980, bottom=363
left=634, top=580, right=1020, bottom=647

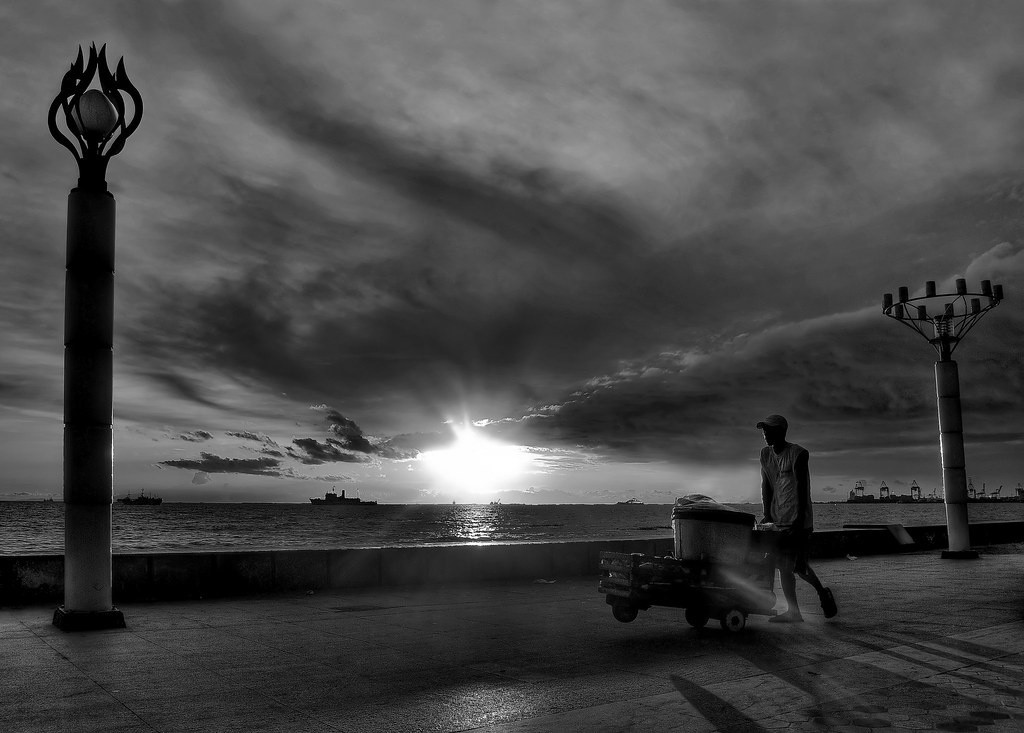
left=756, top=414, right=788, bottom=429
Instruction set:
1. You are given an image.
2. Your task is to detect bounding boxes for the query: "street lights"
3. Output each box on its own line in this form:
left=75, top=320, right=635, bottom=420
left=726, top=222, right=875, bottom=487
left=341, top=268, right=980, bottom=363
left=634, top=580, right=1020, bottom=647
left=884, top=279, right=1004, bottom=561
left=47, top=42, right=144, bottom=631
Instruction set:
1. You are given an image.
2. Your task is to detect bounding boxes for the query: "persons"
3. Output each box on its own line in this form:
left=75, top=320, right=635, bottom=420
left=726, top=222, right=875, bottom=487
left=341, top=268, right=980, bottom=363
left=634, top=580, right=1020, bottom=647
left=756, top=414, right=838, bottom=623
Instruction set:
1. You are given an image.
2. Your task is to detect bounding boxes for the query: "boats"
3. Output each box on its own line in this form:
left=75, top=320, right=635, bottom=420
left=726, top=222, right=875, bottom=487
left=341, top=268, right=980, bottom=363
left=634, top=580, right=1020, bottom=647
left=116, top=489, right=163, bottom=504
left=309, top=485, right=377, bottom=506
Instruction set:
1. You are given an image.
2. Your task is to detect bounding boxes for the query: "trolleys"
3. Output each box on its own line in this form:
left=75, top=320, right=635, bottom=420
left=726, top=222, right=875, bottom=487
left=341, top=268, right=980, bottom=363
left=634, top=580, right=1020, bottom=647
left=599, top=523, right=797, bottom=633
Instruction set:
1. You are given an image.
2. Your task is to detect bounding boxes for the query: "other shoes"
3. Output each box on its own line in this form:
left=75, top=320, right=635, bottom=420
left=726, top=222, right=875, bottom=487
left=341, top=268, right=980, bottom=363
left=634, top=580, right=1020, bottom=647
left=768, top=610, right=804, bottom=623
left=819, top=587, right=838, bottom=618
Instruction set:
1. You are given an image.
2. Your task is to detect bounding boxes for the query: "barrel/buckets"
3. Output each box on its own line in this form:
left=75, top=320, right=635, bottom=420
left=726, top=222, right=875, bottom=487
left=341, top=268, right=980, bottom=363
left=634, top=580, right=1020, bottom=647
left=671, top=507, right=756, bottom=565
